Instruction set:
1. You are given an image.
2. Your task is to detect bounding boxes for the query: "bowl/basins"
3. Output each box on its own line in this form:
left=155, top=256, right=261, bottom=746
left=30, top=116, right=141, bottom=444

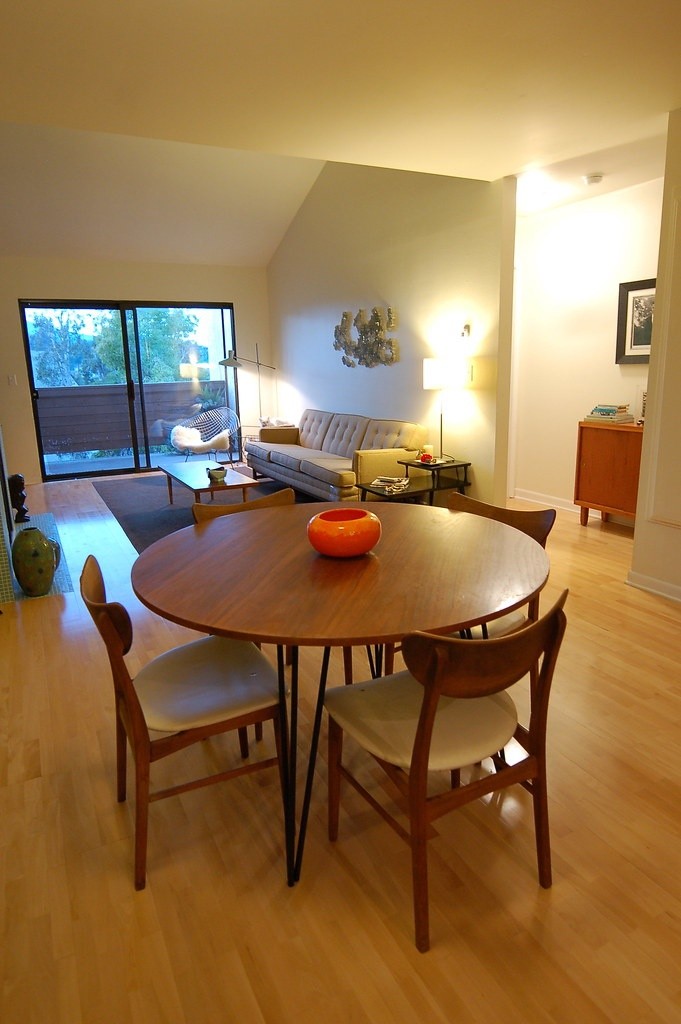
left=307, top=508, right=380, bottom=558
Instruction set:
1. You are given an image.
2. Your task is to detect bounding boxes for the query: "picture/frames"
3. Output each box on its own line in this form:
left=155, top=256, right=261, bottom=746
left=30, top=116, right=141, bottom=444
left=615, top=277, right=656, bottom=365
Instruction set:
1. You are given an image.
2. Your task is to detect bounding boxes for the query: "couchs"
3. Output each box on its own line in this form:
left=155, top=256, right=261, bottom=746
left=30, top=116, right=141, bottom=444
left=246, top=409, right=433, bottom=502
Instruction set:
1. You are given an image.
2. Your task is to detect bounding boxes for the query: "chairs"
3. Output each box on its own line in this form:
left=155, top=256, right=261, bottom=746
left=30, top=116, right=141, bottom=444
left=323, top=587, right=573, bottom=953
left=171, top=406, right=241, bottom=462
left=78, top=554, right=293, bottom=893
left=385, top=492, right=556, bottom=673
left=191, top=488, right=353, bottom=759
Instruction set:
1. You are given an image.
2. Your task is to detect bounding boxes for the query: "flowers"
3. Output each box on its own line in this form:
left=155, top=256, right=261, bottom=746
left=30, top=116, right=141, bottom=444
left=420, top=454, right=437, bottom=464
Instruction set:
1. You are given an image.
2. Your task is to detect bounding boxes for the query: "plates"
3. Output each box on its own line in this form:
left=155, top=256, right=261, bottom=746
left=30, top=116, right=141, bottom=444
left=415, top=460, right=446, bottom=465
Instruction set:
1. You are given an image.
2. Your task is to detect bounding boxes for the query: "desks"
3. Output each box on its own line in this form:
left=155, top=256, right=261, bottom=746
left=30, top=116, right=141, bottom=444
left=354, top=475, right=472, bottom=506
left=398, top=459, right=471, bottom=484
left=130, top=501, right=551, bottom=882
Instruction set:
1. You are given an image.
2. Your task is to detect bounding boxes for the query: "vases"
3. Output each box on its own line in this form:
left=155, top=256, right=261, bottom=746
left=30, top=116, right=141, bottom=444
left=11, top=526, right=61, bottom=597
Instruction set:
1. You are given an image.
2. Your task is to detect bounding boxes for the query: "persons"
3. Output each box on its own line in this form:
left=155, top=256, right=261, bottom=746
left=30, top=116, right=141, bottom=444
left=645, top=302, right=654, bottom=345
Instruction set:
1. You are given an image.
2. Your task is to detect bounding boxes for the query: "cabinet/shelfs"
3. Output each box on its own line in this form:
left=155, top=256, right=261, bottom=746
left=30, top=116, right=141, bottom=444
left=573, top=420, right=644, bottom=527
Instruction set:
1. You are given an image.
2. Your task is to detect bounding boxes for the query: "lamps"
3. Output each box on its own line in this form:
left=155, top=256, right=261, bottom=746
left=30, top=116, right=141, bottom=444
left=218, top=343, right=276, bottom=425
left=421, top=357, right=471, bottom=463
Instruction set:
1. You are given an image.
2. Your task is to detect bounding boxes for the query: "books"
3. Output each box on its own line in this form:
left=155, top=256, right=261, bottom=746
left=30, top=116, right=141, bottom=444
left=584, top=404, right=635, bottom=424
left=370, top=475, right=410, bottom=491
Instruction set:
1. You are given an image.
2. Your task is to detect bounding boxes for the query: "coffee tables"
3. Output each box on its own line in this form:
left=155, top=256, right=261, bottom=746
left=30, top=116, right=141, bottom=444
left=158, top=460, right=260, bottom=505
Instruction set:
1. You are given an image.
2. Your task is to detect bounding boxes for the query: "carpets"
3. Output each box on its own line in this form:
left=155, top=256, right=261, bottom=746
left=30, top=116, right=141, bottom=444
left=93, top=466, right=322, bottom=556
left=12, top=512, right=75, bottom=602
